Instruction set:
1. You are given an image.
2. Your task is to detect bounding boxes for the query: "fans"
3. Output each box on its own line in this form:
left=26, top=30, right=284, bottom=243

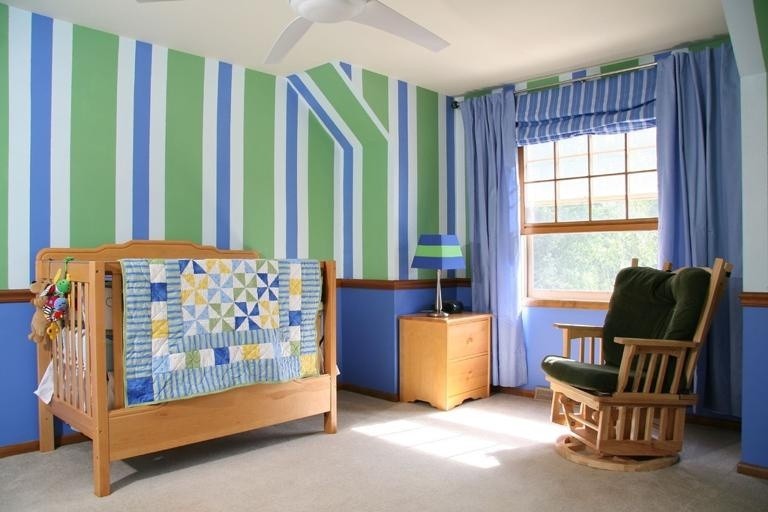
left=261, top=0, right=451, bottom=66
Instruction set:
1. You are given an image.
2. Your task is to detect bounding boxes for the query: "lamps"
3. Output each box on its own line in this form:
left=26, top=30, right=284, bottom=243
left=408, top=230, right=467, bottom=320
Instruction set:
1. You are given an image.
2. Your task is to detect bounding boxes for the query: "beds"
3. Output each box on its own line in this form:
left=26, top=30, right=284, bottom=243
left=35, top=238, right=338, bottom=499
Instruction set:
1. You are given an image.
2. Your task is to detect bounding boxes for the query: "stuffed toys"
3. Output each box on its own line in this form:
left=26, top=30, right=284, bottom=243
left=40, top=279, right=72, bottom=341
left=28, top=276, right=52, bottom=348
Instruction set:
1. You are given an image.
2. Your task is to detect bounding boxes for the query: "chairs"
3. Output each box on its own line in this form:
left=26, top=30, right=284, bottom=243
left=532, top=254, right=731, bottom=473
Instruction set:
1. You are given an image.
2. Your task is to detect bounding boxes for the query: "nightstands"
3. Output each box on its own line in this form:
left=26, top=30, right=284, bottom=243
left=395, top=310, right=495, bottom=412
